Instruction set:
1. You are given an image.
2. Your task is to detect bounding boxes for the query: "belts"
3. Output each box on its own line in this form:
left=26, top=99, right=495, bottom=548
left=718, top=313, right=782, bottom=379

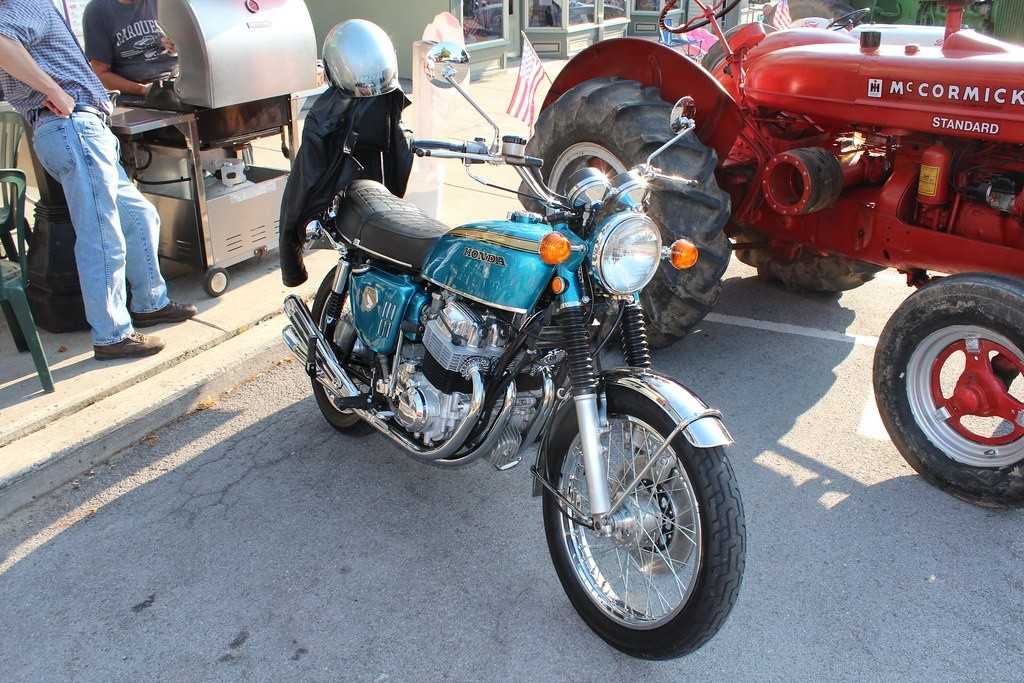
left=31, top=104, right=112, bottom=127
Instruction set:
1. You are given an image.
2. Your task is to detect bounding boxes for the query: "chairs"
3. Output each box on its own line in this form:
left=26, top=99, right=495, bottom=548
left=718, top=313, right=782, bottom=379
left=0, top=105, right=55, bottom=394
left=679, top=24, right=720, bottom=59
left=659, top=18, right=704, bottom=63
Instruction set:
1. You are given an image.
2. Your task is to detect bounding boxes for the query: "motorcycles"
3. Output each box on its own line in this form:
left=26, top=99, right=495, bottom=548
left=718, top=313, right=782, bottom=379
left=278, top=92, right=749, bottom=664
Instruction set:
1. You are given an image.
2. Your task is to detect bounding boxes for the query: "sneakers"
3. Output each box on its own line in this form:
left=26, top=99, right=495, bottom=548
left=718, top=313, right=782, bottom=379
left=94, top=331, right=166, bottom=361
left=131, top=299, right=197, bottom=328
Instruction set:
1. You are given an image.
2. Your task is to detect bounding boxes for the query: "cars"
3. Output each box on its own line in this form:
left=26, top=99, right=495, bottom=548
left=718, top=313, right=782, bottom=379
left=474, top=0, right=624, bottom=33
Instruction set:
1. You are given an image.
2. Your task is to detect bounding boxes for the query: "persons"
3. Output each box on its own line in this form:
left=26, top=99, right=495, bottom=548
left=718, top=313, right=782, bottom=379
left=83, top=0, right=178, bottom=103
left=1, top=0, right=198, bottom=361
left=763, top=4, right=773, bottom=18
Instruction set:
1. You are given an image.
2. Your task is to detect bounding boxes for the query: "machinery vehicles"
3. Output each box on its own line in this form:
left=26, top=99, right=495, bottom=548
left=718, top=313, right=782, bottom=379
left=505, top=0, right=1024, bottom=513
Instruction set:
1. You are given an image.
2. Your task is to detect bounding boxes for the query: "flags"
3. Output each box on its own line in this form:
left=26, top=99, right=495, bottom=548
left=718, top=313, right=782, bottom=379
left=771, top=0, right=792, bottom=30
left=505, top=36, right=545, bottom=139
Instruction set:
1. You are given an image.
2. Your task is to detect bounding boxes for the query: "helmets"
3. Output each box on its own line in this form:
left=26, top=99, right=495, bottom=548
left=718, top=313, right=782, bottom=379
left=322, top=19, right=398, bottom=97
